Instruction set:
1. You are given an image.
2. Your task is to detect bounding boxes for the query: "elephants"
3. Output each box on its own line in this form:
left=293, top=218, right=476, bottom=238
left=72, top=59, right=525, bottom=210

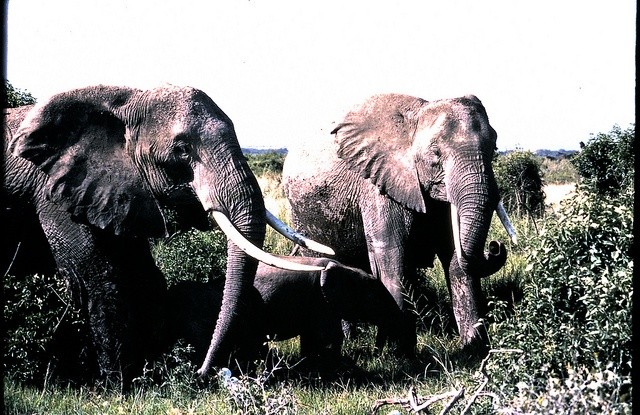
left=0, top=85, right=335, bottom=399
left=284, top=94, right=519, bottom=352
left=250, top=257, right=419, bottom=379
